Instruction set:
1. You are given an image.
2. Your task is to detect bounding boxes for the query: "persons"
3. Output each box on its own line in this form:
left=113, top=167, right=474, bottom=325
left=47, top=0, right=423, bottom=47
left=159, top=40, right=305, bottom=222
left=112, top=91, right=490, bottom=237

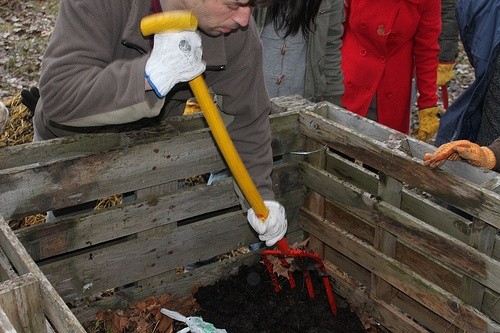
left=250, top=1, right=344, bottom=107
left=423, top=0, right=500, bottom=171
left=33, top=1, right=323, bottom=248
left=341, top=0, right=444, bottom=142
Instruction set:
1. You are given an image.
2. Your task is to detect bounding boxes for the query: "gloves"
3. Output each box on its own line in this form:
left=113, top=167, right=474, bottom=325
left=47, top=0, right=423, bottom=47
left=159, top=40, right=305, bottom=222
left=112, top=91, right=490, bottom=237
left=436, top=63, right=456, bottom=87
left=423, top=140, right=496, bottom=170
left=414, top=107, right=442, bottom=141
left=247, top=200, right=288, bottom=246
left=144, top=31, right=206, bottom=99
